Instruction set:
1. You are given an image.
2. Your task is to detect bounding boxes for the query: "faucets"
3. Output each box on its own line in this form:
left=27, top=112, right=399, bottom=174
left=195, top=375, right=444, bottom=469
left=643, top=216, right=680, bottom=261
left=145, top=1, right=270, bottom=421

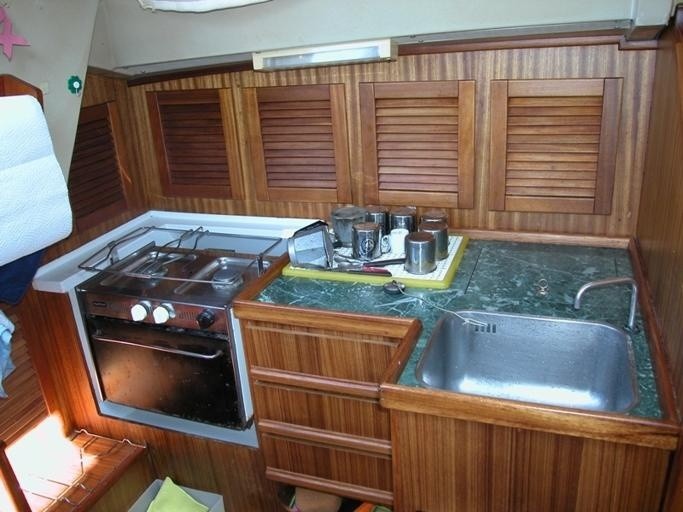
left=573, top=278, right=640, bottom=330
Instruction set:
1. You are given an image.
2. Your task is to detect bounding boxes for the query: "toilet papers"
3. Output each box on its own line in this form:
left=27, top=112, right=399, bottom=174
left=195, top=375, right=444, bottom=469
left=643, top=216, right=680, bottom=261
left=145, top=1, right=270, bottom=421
left=0, top=91, right=72, bottom=267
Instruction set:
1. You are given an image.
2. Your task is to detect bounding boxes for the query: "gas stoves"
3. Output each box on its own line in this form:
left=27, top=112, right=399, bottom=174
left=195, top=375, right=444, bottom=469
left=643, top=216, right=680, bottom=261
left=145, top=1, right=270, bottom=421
left=71, top=240, right=287, bottom=306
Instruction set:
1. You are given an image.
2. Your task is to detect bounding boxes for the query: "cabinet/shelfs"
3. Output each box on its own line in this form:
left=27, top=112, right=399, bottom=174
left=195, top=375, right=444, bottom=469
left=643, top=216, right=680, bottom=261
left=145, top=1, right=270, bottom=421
left=241, top=323, right=395, bottom=508
left=390, top=408, right=679, bottom=512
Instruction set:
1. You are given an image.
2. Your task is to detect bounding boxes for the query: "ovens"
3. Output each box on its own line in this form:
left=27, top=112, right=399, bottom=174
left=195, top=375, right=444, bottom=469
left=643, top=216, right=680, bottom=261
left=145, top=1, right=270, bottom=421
left=86, top=317, right=242, bottom=430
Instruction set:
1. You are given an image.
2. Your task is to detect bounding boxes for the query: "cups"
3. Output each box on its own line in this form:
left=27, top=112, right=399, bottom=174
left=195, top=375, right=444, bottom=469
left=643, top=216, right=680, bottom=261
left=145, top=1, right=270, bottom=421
left=350, top=222, right=382, bottom=262
left=402, top=230, right=437, bottom=276
left=366, top=206, right=450, bottom=262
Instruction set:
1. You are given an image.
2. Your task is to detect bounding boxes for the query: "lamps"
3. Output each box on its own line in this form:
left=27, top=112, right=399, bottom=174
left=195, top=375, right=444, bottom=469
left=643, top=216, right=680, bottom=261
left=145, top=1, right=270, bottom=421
left=252, top=39, right=399, bottom=73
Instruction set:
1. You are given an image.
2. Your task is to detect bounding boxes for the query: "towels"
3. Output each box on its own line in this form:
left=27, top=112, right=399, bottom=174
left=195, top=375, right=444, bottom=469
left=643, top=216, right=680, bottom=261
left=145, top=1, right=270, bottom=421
left=0, top=308, right=16, bottom=399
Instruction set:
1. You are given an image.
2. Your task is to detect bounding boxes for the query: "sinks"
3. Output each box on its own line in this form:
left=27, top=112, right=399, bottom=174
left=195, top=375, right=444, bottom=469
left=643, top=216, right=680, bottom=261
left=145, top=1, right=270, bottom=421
left=415, top=312, right=638, bottom=414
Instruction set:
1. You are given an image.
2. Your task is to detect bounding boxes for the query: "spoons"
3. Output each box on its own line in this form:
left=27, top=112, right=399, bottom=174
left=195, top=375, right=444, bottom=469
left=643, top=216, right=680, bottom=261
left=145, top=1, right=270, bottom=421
left=337, top=258, right=404, bottom=270
